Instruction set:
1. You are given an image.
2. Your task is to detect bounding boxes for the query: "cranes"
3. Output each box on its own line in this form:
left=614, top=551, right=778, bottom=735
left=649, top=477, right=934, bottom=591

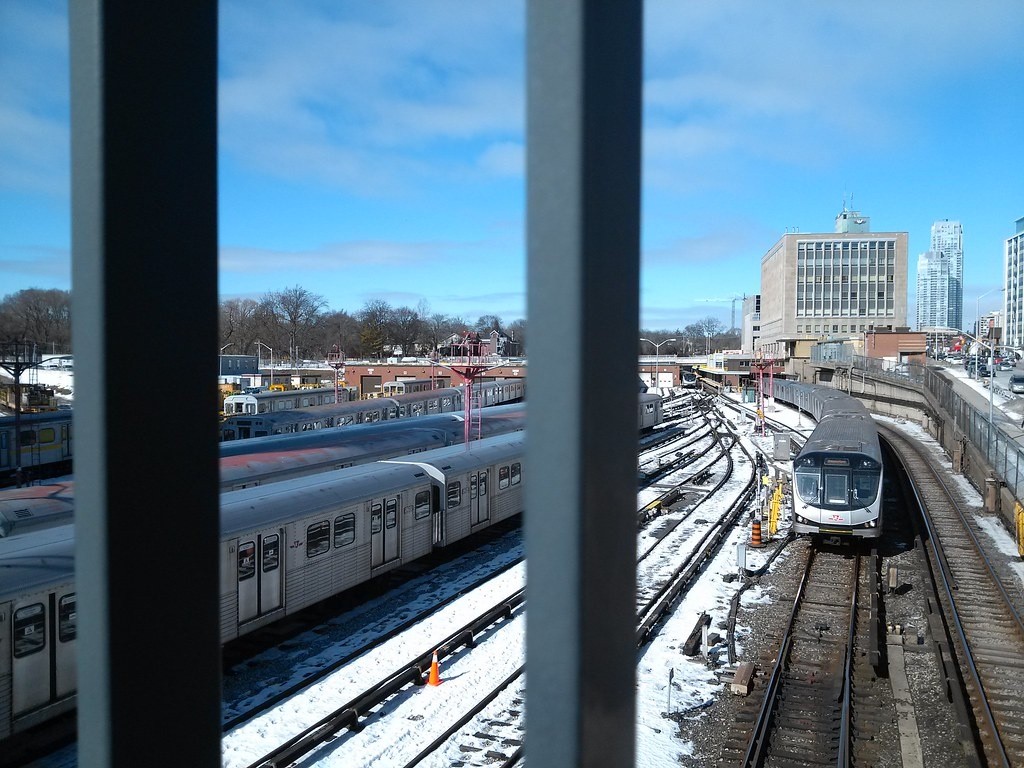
left=694, top=293, right=746, bottom=336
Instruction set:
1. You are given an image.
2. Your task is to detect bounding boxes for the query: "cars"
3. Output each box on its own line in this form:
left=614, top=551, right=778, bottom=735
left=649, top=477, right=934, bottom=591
left=1008, top=374, right=1024, bottom=394
left=937, top=353, right=1017, bottom=377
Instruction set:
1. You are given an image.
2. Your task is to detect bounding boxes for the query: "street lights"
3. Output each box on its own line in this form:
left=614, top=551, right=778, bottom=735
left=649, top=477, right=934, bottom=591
left=219, top=343, right=235, bottom=376
left=934, top=326, right=994, bottom=423
left=935, top=307, right=954, bottom=361
left=640, top=338, right=677, bottom=395
left=254, top=342, right=274, bottom=386
left=974, top=286, right=1006, bottom=380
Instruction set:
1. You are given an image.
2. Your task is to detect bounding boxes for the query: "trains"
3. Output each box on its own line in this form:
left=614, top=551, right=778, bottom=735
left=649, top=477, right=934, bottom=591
left=680, top=369, right=697, bottom=388
left=757, top=377, right=884, bottom=547
left=1, top=379, right=664, bottom=743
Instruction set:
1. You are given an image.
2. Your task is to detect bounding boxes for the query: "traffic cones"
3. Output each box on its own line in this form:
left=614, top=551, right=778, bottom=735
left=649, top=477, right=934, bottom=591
left=425, top=650, right=444, bottom=686
